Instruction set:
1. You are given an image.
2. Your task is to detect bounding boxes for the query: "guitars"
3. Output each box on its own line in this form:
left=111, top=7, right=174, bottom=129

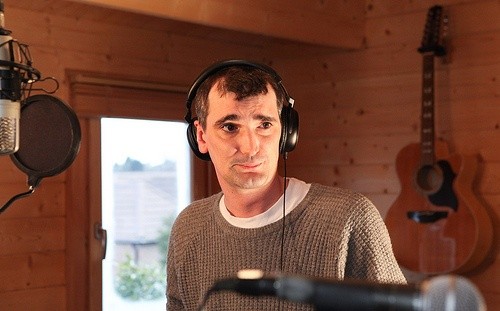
left=385, top=5, right=493, bottom=274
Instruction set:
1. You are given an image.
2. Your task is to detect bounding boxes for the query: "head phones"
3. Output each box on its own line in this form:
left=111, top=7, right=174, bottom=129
left=185, top=58, right=299, bottom=161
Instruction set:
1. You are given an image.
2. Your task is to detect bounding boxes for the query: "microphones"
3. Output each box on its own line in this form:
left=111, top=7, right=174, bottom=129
left=0, top=34, right=24, bottom=155
left=210, top=268, right=487, bottom=311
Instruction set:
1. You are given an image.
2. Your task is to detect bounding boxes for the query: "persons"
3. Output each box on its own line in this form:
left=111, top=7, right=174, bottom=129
left=166, top=65, right=408, bottom=311
